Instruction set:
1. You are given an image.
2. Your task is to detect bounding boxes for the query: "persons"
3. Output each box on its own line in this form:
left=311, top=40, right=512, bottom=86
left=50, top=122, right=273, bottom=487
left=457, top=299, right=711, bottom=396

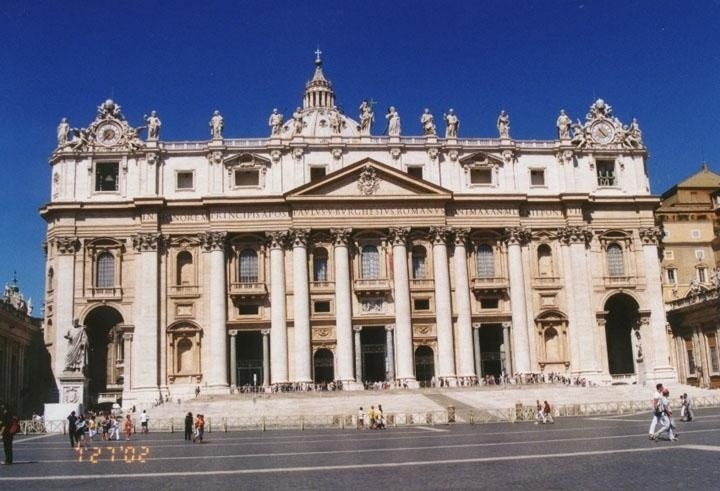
left=647, top=384, right=693, bottom=441
left=184, top=411, right=206, bottom=443
left=270, top=381, right=343, bottom=392
left=230, top=382, right=265, bottom=393
left=0, top=401, right=18, bottom=466
left=358, top=403, right=386, bottom=430
left=544, top=399, right=554, bottom=424
left=535, top=400, right=546, bottom=424
left=439, top=372, right=570, bottom=387
left=66, top=405, right=150, bottom=447
left=365, top=378, right=408, bottom=391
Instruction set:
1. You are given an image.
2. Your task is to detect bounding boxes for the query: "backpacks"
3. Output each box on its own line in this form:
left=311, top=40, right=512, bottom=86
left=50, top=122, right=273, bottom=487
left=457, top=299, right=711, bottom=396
left=655, top=403, right=665, bottom=417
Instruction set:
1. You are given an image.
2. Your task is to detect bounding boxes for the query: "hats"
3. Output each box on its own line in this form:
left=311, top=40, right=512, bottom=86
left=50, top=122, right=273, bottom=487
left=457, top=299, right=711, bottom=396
left=663, top=389, right=669, bottom=394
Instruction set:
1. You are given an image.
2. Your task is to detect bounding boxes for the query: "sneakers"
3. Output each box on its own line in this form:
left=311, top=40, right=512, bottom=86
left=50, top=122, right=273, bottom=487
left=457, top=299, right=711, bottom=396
left=649, top=432, right=678, bottom=441
